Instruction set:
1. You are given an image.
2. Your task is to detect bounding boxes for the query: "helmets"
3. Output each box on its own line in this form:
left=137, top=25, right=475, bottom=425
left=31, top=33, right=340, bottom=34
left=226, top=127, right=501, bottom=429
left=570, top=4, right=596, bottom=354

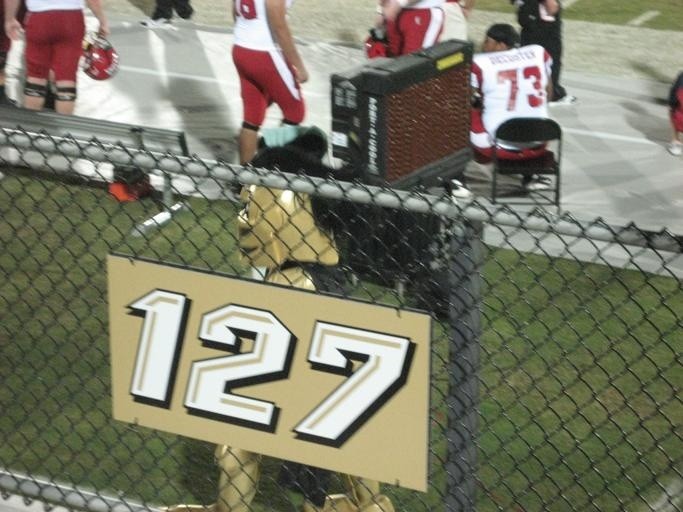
left=363, top=28, right=391, bottom=59
left=81, top=35, right=119, bottom=82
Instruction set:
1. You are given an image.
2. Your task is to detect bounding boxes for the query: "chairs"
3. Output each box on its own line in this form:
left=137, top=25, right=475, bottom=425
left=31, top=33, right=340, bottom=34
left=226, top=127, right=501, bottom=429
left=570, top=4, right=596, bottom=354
left=488, top=117, right=562, bottom=205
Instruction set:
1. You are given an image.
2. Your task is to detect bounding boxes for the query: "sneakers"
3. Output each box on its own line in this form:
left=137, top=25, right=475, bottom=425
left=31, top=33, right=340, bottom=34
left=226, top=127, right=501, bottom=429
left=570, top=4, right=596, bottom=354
left=668, top=134, right=682, bottom=157
left=549, top=92, right=579, bottom=107
left=523, top=175, right=551, bottom=191
left=452, top=178, right=471, bottom=200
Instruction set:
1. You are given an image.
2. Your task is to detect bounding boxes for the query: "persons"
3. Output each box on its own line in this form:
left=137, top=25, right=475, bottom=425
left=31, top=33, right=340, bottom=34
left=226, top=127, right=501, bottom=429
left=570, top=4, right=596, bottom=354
left=375, top=1, right=467, bottom=57
left=227, top=0, right=310, bottom=168
left=204, top=144, right=393, bottom=511
left=2, top=0, right=112, bottom=117
left=662, top=72, right=682, bottom=159
left=1, top=1, right=58, bottom=113
left=508, top=1, right=577, bottom=108
left=467, top=23, right=558, bottom=182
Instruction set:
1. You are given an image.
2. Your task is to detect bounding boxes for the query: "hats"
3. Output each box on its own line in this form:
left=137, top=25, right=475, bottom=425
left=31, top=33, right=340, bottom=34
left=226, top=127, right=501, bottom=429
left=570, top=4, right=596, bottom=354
left=487, top=24, right=523, bottom=50
